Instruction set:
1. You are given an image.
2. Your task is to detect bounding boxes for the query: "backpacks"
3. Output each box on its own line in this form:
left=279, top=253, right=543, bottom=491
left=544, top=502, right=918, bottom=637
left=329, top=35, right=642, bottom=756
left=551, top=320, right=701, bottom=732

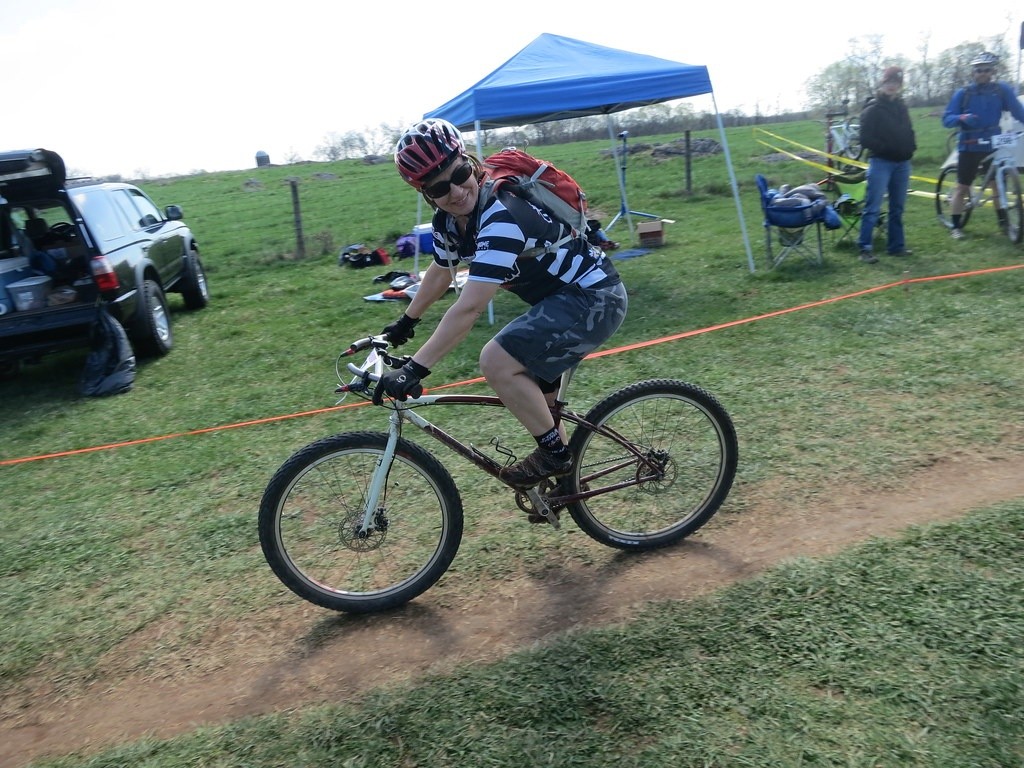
left=479, top=146, right=591, bottom=260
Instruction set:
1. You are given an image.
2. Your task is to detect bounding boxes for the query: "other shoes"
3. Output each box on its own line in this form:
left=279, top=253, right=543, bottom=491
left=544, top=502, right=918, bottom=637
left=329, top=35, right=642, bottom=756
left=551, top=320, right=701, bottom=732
left=1000, top=221, right=1020, bottom=232
left=890, top=250, right=914, bottom=257
left=950, top=229, right=965, bottom=240
left=859, top=250, right=879, bottom=264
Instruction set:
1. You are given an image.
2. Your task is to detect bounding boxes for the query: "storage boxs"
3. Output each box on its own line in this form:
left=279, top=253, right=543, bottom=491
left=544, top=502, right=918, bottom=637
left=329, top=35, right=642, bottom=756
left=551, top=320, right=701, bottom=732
left=5, top=275, right=53, bottom=311
left=0, top=255, right=32, bottom=316
left=413, top=222, right=435, bottom=254
left=636, top=218, right=677, bottom=247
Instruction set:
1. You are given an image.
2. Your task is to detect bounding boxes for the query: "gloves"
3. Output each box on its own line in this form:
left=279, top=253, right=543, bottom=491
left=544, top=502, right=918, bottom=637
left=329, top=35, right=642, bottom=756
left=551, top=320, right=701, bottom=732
left=381, top=314, right=420, bottom=348
left=958, top=113, right=978, bottom=127
left=372, top=359, right=427, bottom=405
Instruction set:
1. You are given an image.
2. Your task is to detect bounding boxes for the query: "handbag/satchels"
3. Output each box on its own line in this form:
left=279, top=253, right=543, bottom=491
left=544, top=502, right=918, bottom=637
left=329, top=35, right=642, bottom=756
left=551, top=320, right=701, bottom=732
left=394, top=232, right=416, bottom=257
left=339, top=243, right=391, bottom=268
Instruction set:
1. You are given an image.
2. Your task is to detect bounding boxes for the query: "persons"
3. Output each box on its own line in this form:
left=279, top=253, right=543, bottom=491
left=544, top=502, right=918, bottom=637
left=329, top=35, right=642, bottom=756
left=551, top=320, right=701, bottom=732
left=942, top=52, right=1024, bottom=241
left=371, top=118, right=628, bottom=523
left=857, top=66, right=916, bottom=265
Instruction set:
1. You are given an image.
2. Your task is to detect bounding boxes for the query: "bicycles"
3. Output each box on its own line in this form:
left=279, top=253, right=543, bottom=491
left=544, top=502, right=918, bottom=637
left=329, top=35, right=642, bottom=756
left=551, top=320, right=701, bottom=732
left=257, top=332, right=739, bottom=613
left=814, top=115, right=864, bottom=161
left=935, top=131, right=1024, bottom=243
left=946, top=132, right=960, bottom=153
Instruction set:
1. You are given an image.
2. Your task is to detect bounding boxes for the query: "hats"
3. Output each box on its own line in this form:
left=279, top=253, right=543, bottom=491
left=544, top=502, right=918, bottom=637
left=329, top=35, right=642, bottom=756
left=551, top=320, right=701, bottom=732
left=885, top=67, right=904, bottom=84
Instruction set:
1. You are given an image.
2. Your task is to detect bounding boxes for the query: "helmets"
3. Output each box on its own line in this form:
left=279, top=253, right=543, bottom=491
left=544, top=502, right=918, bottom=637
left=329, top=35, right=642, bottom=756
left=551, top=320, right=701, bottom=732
left=395, top=118, right=465, bottom=189
left=970, top=52, right=999, bottom=67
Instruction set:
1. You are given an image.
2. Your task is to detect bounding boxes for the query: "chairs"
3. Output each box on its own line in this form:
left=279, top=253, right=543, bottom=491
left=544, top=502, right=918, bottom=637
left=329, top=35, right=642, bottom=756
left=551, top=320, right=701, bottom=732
left=827, top=168, right=889, bottom=247
left=755, top=174, right=824, bottom=271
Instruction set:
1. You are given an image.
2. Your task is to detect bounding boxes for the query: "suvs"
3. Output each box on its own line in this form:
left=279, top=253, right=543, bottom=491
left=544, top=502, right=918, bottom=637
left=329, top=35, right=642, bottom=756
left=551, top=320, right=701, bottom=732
left=0, top=149, right=210, bottom=359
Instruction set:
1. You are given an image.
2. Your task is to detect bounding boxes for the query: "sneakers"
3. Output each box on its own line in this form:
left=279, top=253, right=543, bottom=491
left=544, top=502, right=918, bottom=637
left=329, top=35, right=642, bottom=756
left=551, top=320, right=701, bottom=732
left=500, top=447, right=574, bottom=484
left=529, top=480, right=590, bottom=523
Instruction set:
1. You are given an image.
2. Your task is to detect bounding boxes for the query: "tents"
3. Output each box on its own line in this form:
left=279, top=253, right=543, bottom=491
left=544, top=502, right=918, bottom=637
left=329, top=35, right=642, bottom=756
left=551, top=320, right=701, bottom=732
left=414, top=33, right=755, bottom=324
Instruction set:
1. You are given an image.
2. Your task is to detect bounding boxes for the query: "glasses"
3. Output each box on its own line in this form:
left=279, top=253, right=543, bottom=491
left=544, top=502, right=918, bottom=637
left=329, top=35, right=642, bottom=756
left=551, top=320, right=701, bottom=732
left=423, top=162, right=472, bottom=199
left=974, top=67, right=992, bottom=72
